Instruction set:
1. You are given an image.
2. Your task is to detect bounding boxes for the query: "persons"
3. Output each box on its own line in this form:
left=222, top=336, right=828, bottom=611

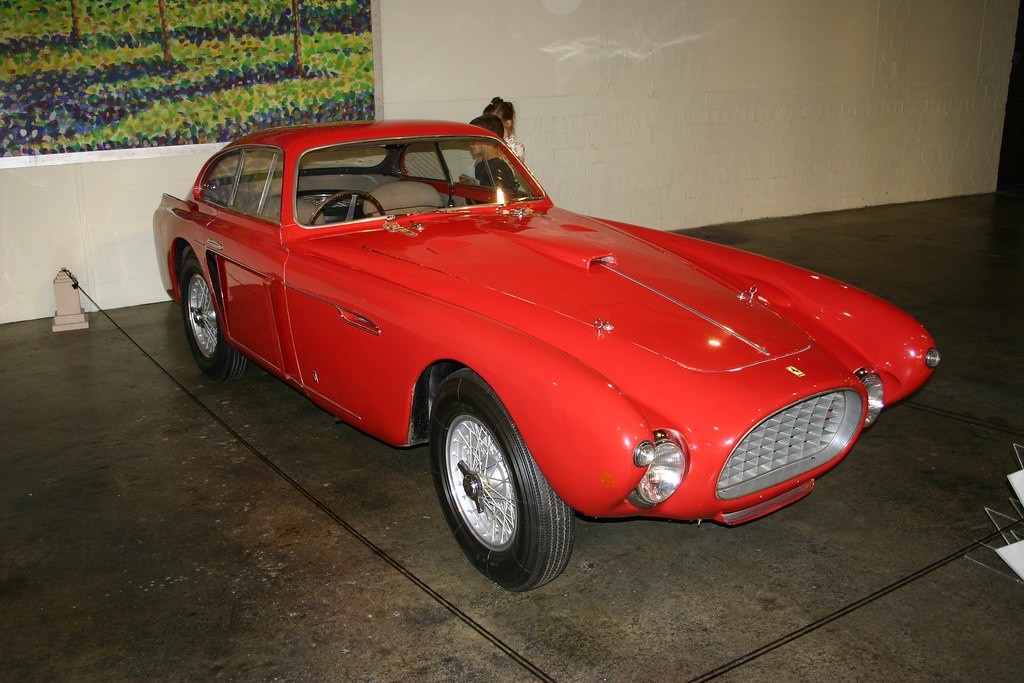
left=468, top=97, right=525, bottom=190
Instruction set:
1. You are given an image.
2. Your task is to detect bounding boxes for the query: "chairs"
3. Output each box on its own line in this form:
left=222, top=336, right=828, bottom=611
left=253, top=197, right=325, bottom=226
left=363, top=180, right=446, bottom=220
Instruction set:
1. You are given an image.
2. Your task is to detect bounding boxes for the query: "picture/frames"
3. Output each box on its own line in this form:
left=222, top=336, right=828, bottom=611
left=0, top=0, right=383, bottom=171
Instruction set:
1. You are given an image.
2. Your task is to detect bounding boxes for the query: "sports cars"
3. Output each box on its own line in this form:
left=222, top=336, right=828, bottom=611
left=155, top=116, right=941, bottom=592
